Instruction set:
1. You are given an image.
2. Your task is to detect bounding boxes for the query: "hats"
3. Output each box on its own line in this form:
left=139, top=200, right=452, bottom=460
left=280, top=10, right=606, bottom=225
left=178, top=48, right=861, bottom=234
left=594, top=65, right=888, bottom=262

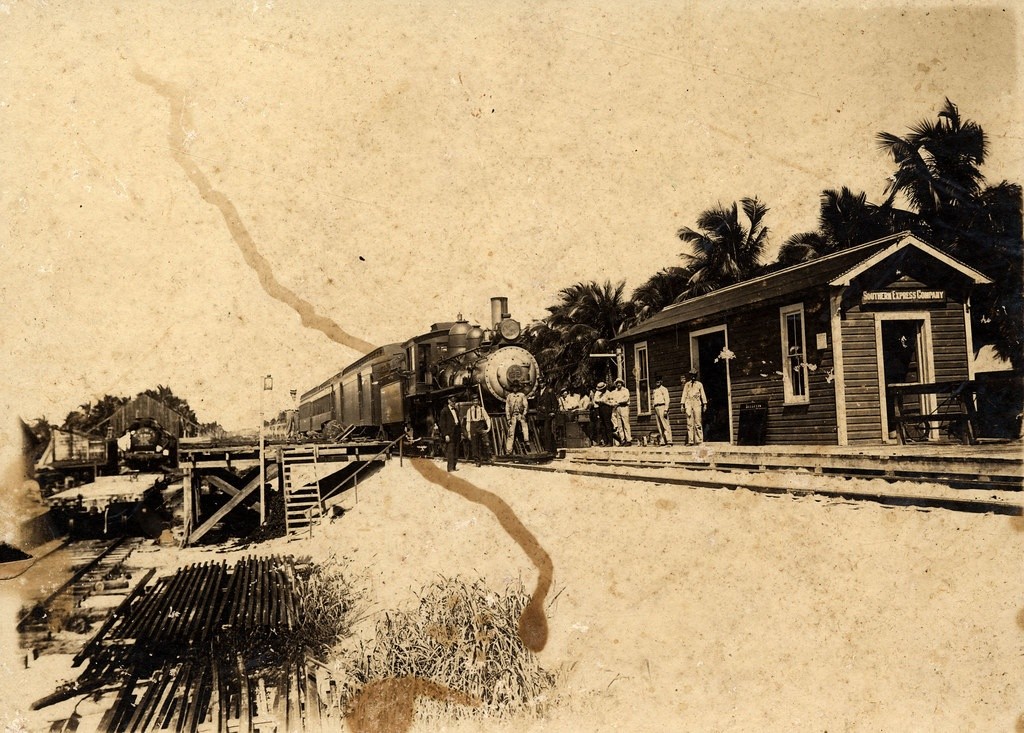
left=449, top=396, right=457, bottom=402
left=560, top=387, right=568, bottom=393
left=596, top=382, right=606, bottom=391
left=538, top=377, right=544, bottom=384
left=614, top=378, right=626, bottom=386
left=688, top=368, right=698, bottom=374
left=510, top=380, right=523, bottom=387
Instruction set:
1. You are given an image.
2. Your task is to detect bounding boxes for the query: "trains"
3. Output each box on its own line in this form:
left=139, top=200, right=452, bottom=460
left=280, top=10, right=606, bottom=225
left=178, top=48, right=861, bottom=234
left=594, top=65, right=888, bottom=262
left=115, top=416, right=178, bottom=475
left=294, top=294, right=565, bottom=470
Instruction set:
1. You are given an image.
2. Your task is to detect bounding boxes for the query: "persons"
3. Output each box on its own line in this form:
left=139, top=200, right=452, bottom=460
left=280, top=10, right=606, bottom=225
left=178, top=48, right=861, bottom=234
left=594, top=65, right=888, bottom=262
left=679, top=367, right=708, bottom=446
left=652, top=374, right=673, bottom=448
left=438, top=369, right=633, bottom=473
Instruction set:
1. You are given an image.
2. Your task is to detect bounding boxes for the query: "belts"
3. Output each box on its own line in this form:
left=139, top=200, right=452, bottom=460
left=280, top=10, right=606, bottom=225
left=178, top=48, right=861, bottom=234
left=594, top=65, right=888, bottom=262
left=654, top=403, right=665, bottom=406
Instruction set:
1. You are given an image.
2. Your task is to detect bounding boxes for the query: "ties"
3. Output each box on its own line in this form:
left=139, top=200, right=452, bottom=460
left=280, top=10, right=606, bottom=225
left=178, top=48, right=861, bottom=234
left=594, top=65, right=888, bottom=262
left=474, top=406, right=477, bottom=419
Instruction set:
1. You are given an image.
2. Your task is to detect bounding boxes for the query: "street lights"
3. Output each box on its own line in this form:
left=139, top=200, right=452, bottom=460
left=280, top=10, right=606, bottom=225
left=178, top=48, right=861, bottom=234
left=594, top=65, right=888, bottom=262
left=257, top=375, right=278, bottom=528
left=291, top=390, right=299, bottom=445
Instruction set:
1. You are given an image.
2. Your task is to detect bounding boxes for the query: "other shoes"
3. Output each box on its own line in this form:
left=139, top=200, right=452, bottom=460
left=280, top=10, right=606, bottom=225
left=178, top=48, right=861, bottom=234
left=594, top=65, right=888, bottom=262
left=475, top=463, right=481, bottom=467
left=686, top=443, right=700, bottom=446
left=660, top=443, right=673, bottom=446
left=618, top=441, right=632, bottom=447
left=448, top=468, right=459, bottom=472
